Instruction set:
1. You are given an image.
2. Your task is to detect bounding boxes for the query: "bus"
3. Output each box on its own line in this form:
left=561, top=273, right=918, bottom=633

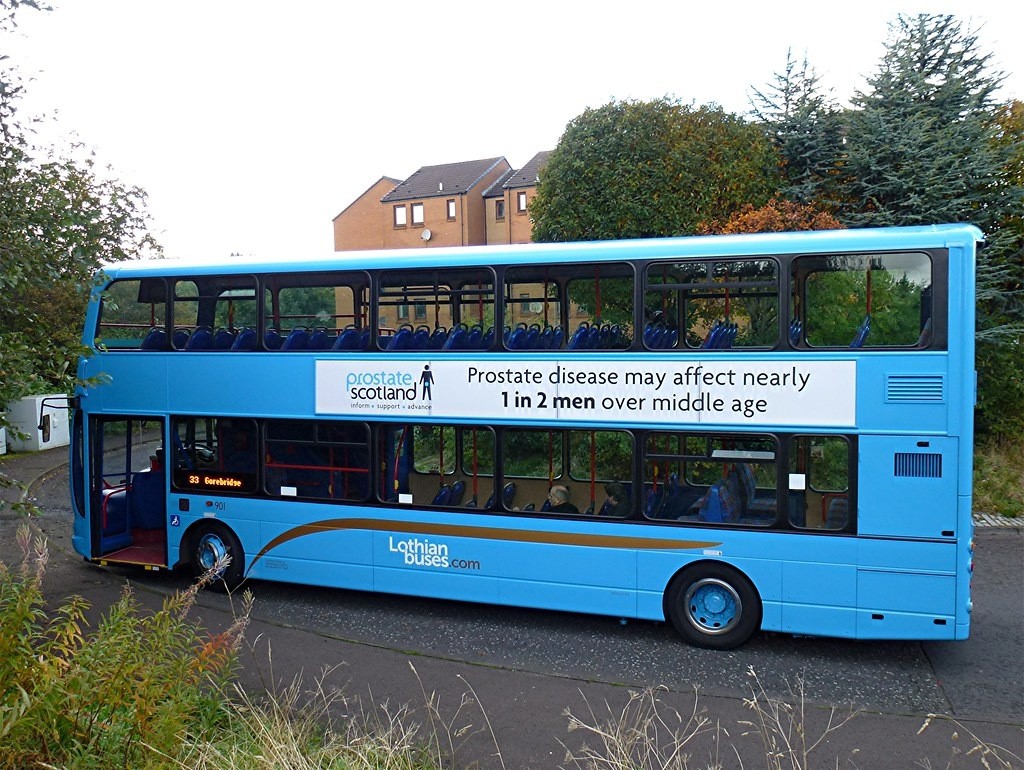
left=37, top=223, right=983, bottom=650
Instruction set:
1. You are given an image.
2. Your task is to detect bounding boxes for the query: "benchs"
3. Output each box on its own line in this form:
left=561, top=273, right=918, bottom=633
left=659, top=461, right=848, bottom=531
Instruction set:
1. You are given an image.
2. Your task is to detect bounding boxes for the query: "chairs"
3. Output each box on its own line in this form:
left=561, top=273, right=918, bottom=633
left=428, top=479, right=612, bottom=514
left=141, top=315, right=872, bottom=351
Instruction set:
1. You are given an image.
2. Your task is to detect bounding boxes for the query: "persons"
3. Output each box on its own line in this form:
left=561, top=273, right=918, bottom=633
left=513, top=485, right=581, bottom=514
left=603, top=482, right=633, bottom=518
